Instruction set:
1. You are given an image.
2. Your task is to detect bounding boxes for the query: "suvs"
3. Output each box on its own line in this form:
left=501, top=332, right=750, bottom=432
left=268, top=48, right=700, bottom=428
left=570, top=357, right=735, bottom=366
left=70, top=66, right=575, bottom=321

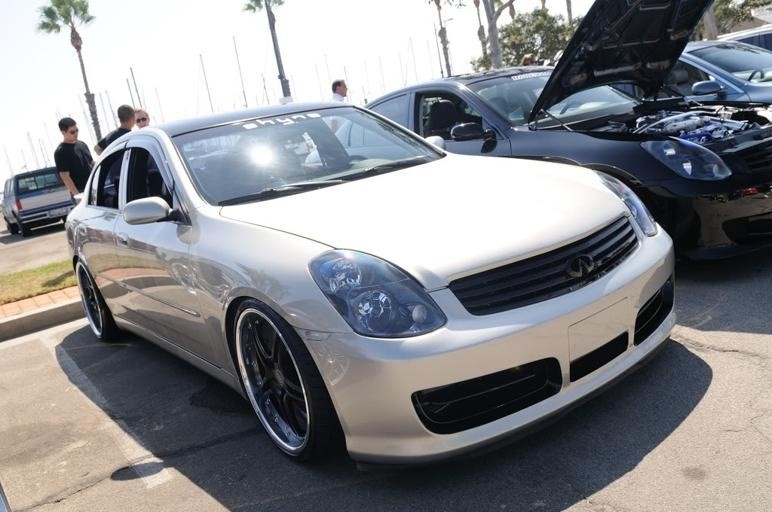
left=1, top=167, right=74, bottom=236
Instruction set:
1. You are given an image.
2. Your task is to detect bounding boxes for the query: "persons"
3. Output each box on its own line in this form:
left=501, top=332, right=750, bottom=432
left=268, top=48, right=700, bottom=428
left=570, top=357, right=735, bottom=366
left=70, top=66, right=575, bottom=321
left=52, top=106, right=151, bottom=206
left=517, top=51, right=539, bottom=67
left=332, top=81, right=348, bottom=134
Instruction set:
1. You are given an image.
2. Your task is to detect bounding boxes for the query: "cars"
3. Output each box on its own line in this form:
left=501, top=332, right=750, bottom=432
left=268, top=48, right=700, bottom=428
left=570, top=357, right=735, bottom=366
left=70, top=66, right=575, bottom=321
left=365, top=0, right=772, bottom=263
left=63, top=99, right=680, bottom=470
left=616, top=38, right=772, bottom=107
left=700, top=23, right=772, bottom=53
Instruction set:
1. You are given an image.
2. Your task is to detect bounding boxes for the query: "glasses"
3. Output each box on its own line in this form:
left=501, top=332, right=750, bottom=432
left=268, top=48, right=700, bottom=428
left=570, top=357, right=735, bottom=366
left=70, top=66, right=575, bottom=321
left=137, top=118, right=146, bottom=123
left=70, top=129, right=78, bottom=134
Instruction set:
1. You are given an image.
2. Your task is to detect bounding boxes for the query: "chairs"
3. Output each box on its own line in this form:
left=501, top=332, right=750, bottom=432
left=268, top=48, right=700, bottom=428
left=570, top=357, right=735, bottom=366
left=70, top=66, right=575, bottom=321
left=664, top=68, right=693, bottom=99
left=481, top=96, right=511, bottom=133
left=421, top=97, right=463, bottom=144
